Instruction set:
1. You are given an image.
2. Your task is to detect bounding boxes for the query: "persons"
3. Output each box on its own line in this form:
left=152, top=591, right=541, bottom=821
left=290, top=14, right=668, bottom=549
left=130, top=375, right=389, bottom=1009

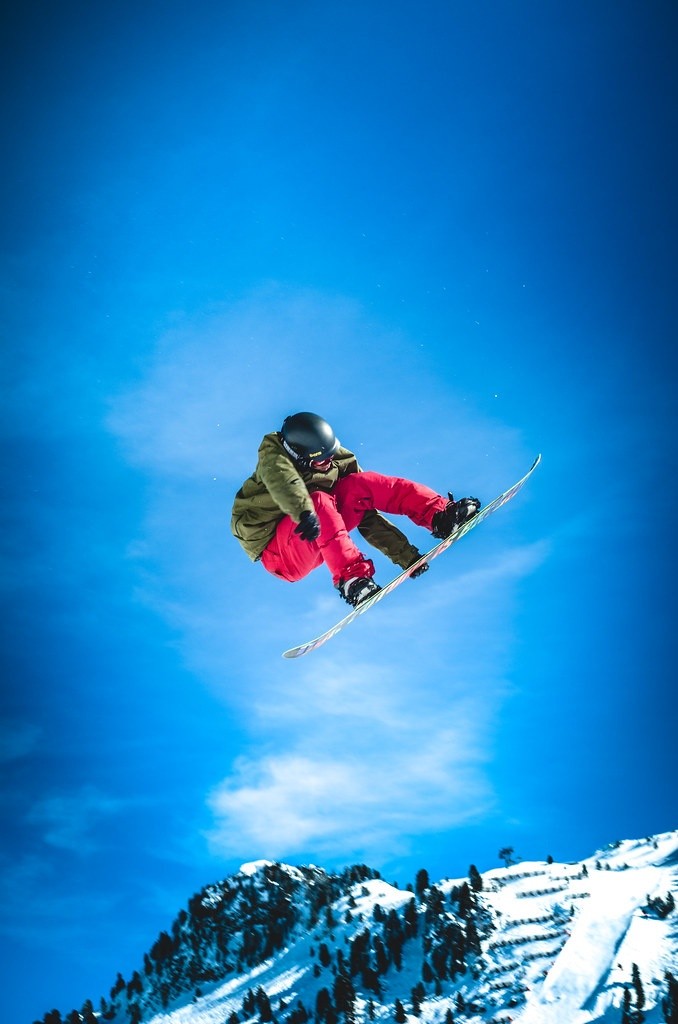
left=230, top=412, right=481, bottom=608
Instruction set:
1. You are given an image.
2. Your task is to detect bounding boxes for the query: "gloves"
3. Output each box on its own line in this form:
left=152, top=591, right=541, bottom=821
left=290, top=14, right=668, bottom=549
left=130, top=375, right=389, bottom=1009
left=407, top=554, right=430, bottom=579
left=293, top=511, right=321, bottom=541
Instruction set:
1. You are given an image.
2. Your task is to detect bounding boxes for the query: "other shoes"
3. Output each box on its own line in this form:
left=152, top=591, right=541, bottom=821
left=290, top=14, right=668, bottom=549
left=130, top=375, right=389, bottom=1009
left=338, top=570, right=381, bottom=609
left=429, top=491, right=482, bottom=541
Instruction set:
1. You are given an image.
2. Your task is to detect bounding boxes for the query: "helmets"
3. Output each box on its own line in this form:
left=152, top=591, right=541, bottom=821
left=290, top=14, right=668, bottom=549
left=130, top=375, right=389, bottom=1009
left=279, top=411, right=340, bottom=467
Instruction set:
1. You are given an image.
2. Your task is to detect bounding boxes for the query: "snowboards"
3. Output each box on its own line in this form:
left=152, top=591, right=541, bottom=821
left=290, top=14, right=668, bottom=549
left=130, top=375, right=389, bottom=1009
left=280, top=452, right=542, bottom=660
